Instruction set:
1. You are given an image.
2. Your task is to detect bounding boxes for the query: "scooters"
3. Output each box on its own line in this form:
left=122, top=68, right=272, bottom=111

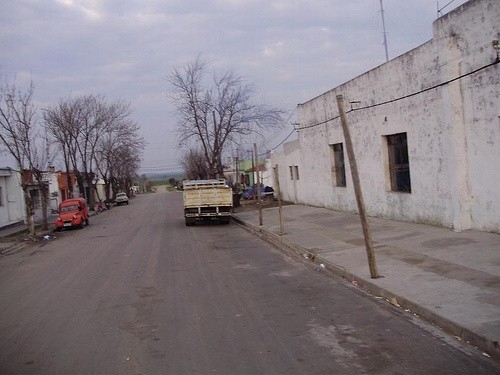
left=104, top=200, right=109, bottom=210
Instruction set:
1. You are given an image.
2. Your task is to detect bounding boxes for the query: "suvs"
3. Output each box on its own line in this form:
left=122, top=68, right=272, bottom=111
left=53, top=197, right=90, bottom=229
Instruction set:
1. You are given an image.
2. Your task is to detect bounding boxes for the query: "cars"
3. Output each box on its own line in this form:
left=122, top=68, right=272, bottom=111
left=114, top=192, right=129, bottom=206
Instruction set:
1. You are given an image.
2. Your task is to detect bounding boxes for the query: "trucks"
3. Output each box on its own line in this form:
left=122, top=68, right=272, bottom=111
left=180, top=178, right=234, bottom=226
